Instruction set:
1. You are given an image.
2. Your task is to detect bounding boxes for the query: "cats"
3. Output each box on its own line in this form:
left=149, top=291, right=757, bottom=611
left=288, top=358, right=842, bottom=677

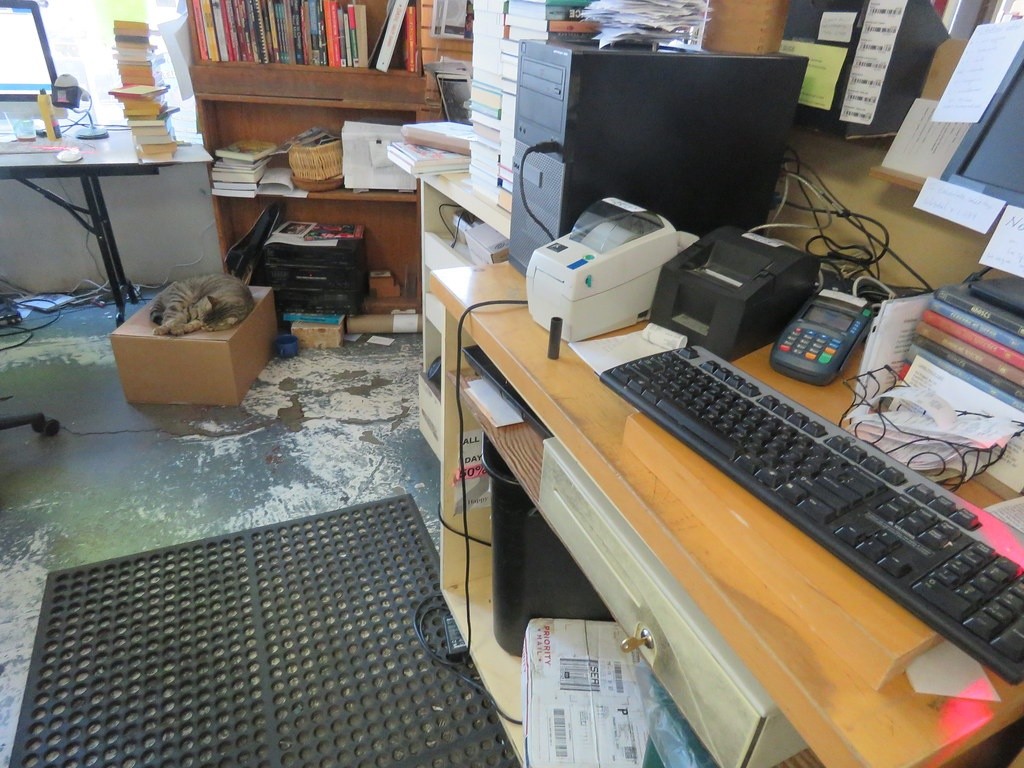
left=150, top=272, right=253, bottom=337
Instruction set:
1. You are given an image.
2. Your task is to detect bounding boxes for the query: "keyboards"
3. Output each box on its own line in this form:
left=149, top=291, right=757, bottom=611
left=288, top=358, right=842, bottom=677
left=600, top=346, right=1024, bottom=687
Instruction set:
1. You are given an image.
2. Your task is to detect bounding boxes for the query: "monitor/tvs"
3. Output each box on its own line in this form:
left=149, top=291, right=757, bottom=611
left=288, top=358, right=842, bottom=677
left=941, top=40, right=1024, bottom=316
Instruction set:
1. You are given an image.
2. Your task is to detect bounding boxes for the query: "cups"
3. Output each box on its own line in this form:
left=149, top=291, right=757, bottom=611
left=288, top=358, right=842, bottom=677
left=4, top=109, right=37, bottom=141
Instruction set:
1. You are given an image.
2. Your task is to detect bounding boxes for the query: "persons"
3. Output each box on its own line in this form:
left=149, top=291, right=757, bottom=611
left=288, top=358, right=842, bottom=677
left=318, top=231, right=354, bottom=239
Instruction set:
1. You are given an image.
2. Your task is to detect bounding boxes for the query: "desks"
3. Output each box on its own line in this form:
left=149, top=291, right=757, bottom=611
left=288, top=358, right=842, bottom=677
left=0, top=128, right=162, bottom=328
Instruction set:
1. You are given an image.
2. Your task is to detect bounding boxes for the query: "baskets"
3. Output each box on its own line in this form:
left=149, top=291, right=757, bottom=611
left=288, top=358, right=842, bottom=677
left=290, top=172, right=345, bottom=191
left=287, top=140, right=343, bottom=180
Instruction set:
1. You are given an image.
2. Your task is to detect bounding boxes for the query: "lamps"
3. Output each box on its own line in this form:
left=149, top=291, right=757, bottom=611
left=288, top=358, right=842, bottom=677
left=52, top=73, right=111, bottom=140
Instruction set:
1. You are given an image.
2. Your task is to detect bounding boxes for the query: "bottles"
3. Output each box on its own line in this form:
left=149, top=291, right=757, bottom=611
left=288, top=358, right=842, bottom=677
left=38, top=88, right=62, bottom=141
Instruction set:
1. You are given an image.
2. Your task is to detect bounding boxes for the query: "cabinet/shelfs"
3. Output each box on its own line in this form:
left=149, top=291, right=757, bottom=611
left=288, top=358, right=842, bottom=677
left=186, top=0, right=427, bottom=323
left=417, top=171, right=513, bottom=462
left=429, top=260, right=1024, bottom=768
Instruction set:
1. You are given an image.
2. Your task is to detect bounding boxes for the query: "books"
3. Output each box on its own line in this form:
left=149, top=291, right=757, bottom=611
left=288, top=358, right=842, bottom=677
left=887, top=274, right=1024, bottom=500
left=283, top=313, right=345, bottom=324
left=111, top=0, right=601, bottom=215
left=302, top=224, right=365, bottom=240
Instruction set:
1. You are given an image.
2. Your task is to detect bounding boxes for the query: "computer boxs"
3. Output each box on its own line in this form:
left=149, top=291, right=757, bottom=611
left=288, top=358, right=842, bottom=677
left=509, top=40, right=810, bottom=279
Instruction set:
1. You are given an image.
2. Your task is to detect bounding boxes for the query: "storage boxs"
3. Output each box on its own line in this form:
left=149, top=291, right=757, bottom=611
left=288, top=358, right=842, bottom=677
left=291, top=321, right=344, bottom=349
left=110, top=283, right=279, bottom=408
